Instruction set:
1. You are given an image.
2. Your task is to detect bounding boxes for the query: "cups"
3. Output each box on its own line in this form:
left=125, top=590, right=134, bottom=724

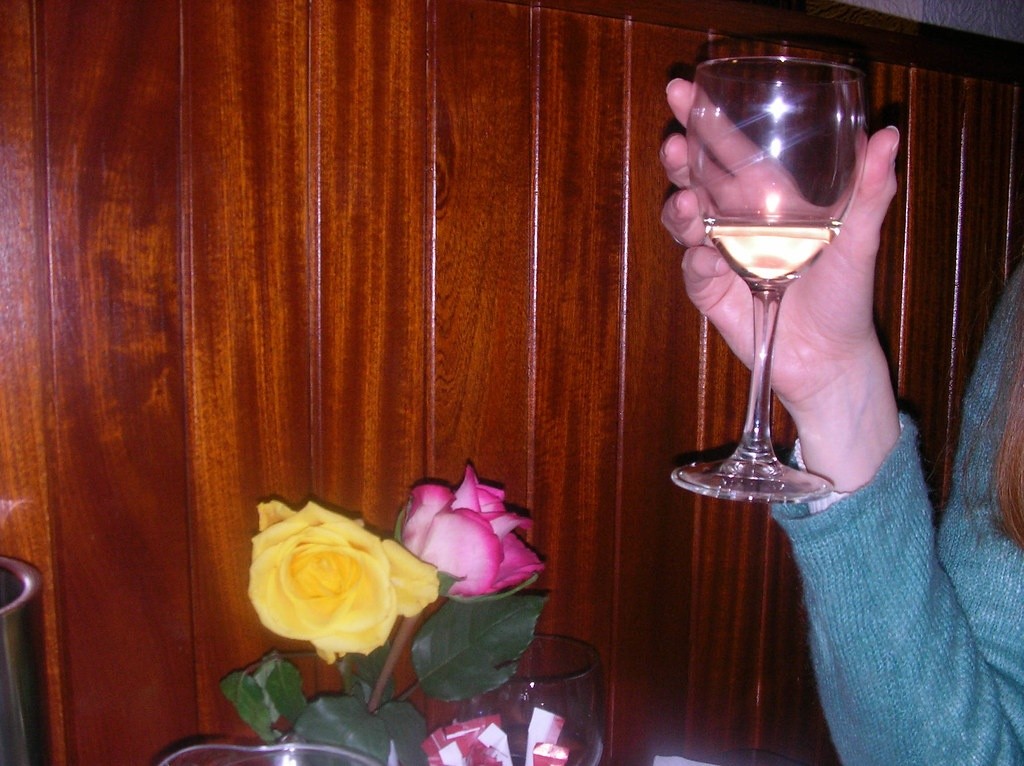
left=158, top=746, right=381, bottom=766
left=0, top=556, right=49, bottom=766
left=433, top=635, right=599, bottom=766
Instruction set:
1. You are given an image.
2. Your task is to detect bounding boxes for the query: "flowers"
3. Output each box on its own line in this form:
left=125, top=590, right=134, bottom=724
left=221, top=464, right=551, bottom=766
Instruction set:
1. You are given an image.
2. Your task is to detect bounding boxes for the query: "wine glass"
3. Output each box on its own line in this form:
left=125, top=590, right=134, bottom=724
left=670, top=58, right=866, bottom=497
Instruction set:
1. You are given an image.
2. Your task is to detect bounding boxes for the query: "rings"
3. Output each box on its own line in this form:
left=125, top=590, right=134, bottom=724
left=672, top=230, right=710, bottom=248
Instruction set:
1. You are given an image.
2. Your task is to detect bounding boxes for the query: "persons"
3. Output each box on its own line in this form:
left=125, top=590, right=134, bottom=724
left=658, top=73, right=1024, bottom=765
